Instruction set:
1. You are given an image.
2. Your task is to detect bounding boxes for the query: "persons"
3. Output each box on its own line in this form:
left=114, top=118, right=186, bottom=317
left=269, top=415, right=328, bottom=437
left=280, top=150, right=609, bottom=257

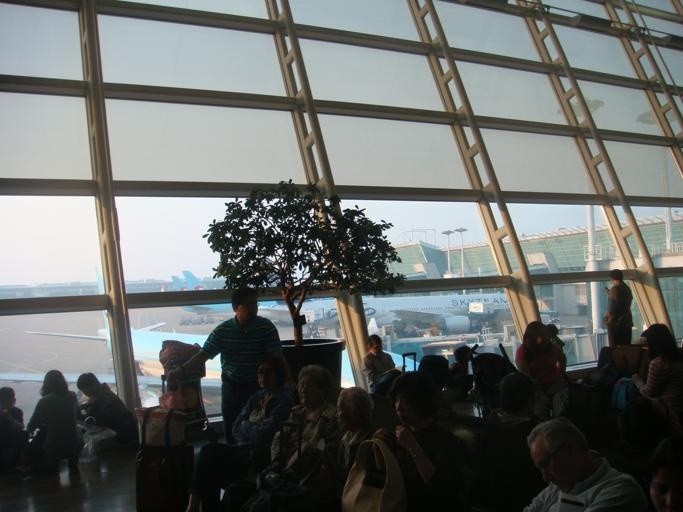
left=24, top=370, right=87, bottom=485
left=0, top=386, right=26, bottom=472
left=603, top=269, right=633, bottom=346
left=76, top=371, right=138, bottom=456
left=186, top=320, right=683, bottom=511
left=164, top=288, right=296, bottom=445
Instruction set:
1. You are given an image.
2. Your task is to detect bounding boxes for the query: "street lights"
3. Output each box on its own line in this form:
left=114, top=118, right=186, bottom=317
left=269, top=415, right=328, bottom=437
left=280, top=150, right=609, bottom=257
left=454, top=228, right=468, bottom=277
left=637, top=107, right=678, bottom=253
left=442, top=230, right=455, bottom=276
left=558, top=98, right=608, bottom=333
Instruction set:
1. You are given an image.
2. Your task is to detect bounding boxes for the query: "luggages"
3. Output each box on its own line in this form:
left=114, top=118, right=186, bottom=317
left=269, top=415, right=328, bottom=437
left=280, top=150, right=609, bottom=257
left=136, top=376, right=194, bottom=512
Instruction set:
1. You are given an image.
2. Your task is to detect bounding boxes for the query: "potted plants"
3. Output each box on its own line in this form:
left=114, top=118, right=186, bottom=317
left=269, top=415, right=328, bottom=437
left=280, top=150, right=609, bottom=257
left=201, top=179, right=407, bottom=407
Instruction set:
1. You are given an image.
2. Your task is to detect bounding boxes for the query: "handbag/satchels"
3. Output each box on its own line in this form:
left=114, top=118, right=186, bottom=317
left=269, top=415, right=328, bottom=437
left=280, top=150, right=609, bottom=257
left=134, top=341, right=205, bottom=447
left=342, top=438, right=404, bottom=512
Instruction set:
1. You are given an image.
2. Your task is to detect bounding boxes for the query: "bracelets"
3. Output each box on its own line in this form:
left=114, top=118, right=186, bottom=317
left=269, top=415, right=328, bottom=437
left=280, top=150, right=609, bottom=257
left=175, top=366, right=185, bottom=374
